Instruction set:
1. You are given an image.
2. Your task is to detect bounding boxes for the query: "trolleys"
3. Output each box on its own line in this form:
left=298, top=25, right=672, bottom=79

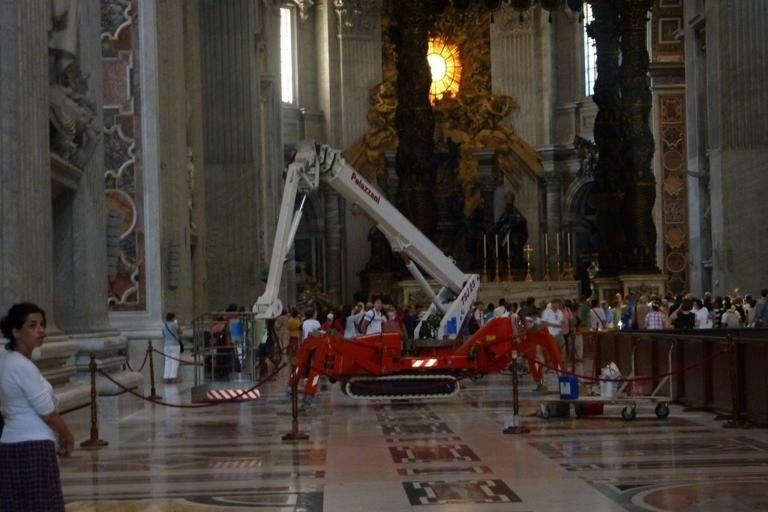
left=537, top=335, right=676, bottom=420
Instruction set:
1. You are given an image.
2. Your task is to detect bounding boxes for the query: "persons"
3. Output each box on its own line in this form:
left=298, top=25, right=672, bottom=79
left=463, top=297, right=580, bottom=373
left=1, top=302, right=76, bottom=510
left=580, top=287, right=768, bottom=333
left=220, top=298, right=420, bottom=369
left=160, top=311, right=184, bottom=384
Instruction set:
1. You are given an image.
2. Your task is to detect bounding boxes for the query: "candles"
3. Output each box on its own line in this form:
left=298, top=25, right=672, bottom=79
left=481, top=232, right=573, bottom=257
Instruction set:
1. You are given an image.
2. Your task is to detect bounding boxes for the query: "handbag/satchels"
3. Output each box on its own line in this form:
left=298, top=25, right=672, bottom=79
left=178, top=337, right=185, bottom=354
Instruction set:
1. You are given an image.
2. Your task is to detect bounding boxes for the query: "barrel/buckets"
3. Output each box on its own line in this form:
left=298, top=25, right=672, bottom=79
left=601, top=380, right=617, bottom=398
left=559, top=375, right=578, bottom=400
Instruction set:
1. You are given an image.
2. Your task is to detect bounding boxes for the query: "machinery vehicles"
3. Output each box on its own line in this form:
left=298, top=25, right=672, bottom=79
left=252, top=136, right=572, bottom=414
